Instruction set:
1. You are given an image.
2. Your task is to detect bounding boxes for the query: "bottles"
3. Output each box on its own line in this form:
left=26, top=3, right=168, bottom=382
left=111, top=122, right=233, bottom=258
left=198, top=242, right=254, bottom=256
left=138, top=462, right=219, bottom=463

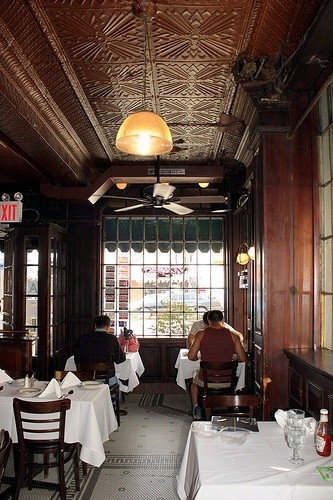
left=124, top=344, right=128, bottom=353
left=316, top=409, right=331, bottom=456
left=24, top=374, right=34, bottom=387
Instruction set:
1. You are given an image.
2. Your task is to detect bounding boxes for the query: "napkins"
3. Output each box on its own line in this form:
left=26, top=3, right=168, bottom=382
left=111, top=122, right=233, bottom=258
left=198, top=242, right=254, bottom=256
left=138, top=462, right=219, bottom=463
left=0, top=369, right=13, bottom=382
left=40, top=378, right=61, bottom=398
left=59, top=371, right=82, bottom=388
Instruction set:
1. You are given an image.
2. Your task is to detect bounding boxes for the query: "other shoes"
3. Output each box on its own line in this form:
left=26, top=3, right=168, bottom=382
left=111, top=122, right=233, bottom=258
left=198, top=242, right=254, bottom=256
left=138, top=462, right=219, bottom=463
left=193, top=404, right=202, bottom=421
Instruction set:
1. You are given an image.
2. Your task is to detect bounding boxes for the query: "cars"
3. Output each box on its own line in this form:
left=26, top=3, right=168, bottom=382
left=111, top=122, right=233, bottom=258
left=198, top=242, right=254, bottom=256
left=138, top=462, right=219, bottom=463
left=135, top=293, right=223, bottom=318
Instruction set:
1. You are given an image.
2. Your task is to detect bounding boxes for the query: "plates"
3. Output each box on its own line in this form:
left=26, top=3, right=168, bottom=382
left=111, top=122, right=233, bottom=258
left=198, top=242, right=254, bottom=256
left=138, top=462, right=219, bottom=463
left=13, top=378, right=37, bottom=383
left=16, top=388, right=42, bottom=396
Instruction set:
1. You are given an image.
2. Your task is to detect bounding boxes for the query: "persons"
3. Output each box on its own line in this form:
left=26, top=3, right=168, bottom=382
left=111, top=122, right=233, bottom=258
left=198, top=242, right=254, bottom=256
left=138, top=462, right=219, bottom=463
left=187, top=310, right=247, bottom=421
left=55, top=315, right=129, bottom=415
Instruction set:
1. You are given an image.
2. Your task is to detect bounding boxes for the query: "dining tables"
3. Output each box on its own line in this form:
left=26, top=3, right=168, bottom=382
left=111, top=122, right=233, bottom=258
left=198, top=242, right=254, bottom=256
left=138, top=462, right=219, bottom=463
left=0, top=380, right=119, bottom=500
left=64, top=349, right=145, bottom=401
left=177, top=420, right=332, bottom=500
left=175, top=348, right=245, bottom=394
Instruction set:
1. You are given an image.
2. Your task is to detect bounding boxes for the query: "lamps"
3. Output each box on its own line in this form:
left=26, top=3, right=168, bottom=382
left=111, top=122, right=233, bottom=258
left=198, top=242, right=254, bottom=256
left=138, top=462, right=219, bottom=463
left=237, top=242, right=256, bottom=265
left=198, top=182, right=209, bottom=188
left=116, top=182, right=127, bottom=190
left=115, top=0, right=175, bottom=155
left=0, top=192, right=23, bottom=202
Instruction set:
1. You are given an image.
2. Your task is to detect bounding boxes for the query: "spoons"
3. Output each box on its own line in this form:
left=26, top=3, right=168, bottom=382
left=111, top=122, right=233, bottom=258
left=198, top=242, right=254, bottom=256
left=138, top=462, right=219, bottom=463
left=63, top=390, right=73, bottom=400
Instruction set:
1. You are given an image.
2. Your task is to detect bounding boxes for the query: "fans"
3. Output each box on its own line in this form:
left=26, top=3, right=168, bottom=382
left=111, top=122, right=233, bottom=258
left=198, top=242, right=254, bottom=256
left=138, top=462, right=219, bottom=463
left=92, top=155, right=194, bottom=216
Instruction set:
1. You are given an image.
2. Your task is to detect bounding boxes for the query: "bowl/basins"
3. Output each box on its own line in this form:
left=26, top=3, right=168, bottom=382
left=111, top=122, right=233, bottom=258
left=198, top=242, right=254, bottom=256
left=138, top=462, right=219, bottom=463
left=193, top=424, right=250, bottom=445
left=81, top=380, right=103, bottom=388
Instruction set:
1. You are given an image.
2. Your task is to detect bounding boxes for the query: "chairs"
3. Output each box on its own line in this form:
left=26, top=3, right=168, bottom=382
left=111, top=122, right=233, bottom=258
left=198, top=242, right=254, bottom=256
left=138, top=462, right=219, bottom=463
left=200, top=360, right=239, bottom=420
left=202, top=395, right=261, bottom=421
left=55, top=363, right=121, bottom=429
left=12, top=397, right=80, bottom=499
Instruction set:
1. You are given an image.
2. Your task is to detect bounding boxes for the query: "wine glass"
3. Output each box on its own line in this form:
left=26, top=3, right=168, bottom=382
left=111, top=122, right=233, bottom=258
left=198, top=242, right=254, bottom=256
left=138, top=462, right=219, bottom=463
left=284, top=409, right=306, bottom=465
